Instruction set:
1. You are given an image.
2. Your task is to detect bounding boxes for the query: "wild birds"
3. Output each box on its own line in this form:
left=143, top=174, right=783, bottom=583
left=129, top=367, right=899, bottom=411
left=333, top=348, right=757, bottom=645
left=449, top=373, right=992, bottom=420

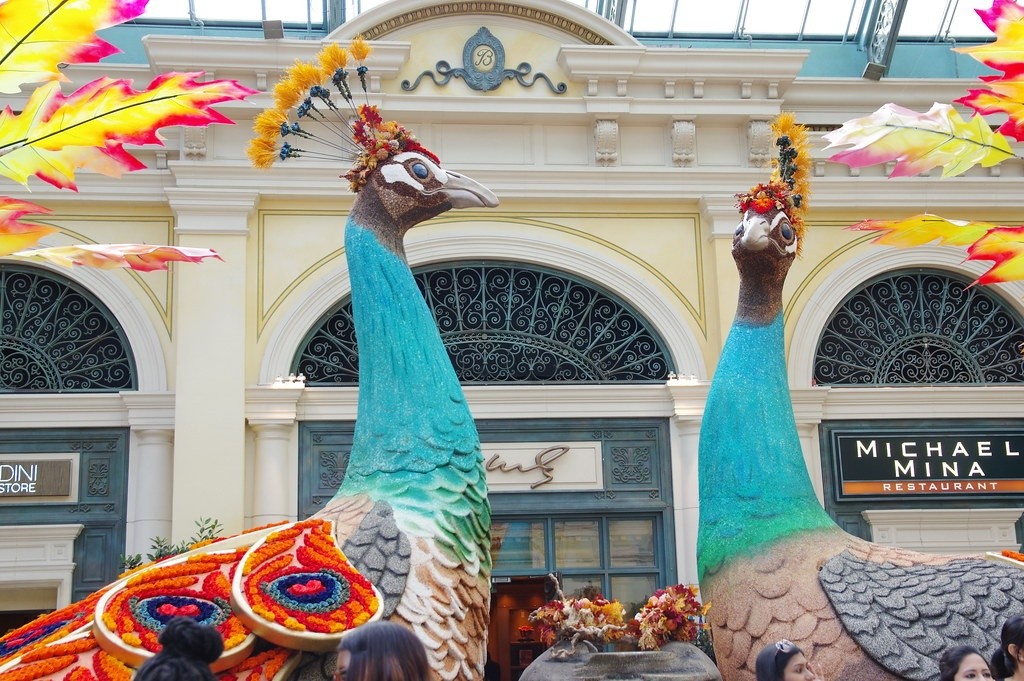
left=696, top=112, right=1024, bottom=681
left=0, top=36, right=499, bottom=681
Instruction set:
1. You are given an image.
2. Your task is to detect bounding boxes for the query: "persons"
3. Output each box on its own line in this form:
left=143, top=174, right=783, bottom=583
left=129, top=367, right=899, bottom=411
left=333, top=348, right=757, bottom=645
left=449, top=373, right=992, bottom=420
left=939, top=644, right=996, bottom=681
left=331, top=622, right=433, bottom=681
left=134, top=616, right=223, bottom=681
left=992, top=612, right=1024, bottom=681
left=753, top=639, right=815, bottom=680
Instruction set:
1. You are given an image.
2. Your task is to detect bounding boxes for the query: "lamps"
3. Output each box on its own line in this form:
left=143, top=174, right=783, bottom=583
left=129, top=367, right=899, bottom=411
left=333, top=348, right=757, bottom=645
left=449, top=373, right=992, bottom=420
left=261, top=20, right=285, bottom=38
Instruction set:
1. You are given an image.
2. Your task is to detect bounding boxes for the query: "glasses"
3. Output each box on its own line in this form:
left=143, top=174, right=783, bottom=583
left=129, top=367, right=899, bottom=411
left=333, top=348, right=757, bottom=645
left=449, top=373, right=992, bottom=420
left=774, top=639, right=796, bottom=671
